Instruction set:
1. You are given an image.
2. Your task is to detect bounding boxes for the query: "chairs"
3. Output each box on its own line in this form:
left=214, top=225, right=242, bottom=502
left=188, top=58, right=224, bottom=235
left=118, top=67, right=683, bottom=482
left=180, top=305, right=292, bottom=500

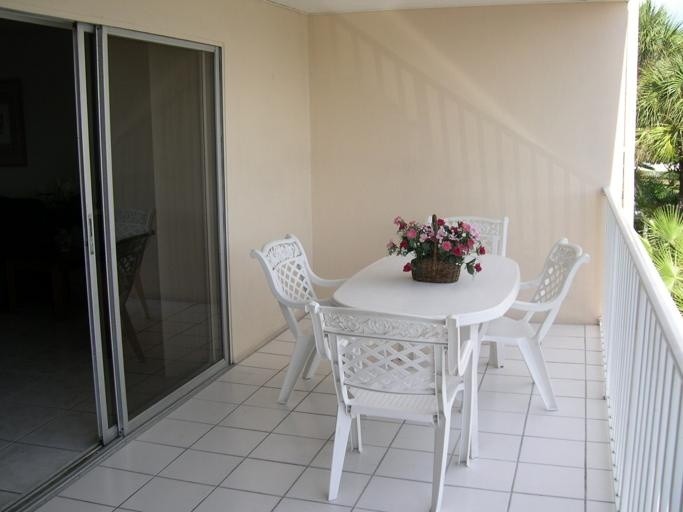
left=117, top=230, right=155, bottom=365
left=306, top=301, right=478, bottom=512
left=116, top=207, right=156, bottom=318
left=427, top=216, right=512, bottom=254
left=252, top=232, right=346, bottom=408
left=467, top=237, right=591, bottom=411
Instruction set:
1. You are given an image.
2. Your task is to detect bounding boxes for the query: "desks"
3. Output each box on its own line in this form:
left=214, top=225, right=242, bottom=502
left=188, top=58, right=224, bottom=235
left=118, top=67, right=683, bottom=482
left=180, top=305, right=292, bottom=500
left=332, top=253, right=522, bottom=326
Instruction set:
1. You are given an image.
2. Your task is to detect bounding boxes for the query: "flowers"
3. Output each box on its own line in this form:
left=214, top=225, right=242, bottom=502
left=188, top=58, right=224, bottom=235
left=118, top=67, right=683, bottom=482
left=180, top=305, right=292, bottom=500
left=386, top=215, right=486, bottom=275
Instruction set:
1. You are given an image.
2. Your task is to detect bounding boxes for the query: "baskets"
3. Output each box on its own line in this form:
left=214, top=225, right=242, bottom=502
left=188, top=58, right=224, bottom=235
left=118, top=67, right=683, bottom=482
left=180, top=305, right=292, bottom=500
left=411, top=255, right=460, bottom=283
